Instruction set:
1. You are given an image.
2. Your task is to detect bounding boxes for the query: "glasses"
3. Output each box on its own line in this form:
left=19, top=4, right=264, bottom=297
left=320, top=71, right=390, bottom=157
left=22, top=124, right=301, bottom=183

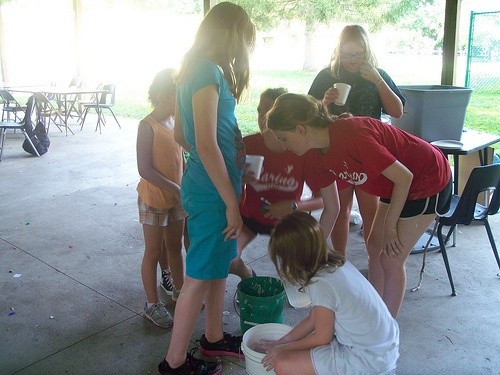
left=342, top=51, right=365, bottom=58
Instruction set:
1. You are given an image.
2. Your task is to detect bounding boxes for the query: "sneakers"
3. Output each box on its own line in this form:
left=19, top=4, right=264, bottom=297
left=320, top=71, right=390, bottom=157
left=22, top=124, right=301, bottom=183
left=171, top=287, right=205, bottom=309
left=143, top=301, right=173, bottom=328
left=160, top=270, right=175, bottom=295
left=198, top=331, right=245, bottom=359
left=157, top=347, right=222, bottom=375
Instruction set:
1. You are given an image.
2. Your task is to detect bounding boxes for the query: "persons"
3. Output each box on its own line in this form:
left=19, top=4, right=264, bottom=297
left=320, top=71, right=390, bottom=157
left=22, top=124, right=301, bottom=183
left=160, top=4, right=261, bottom=375
left=137, top=66, right=206, bottom=329
left=255, top=211, right=400, bottom=375
left=264, top=93, right=452, bottom=325
left=221, top=87, right=326, bottom=305
left=308, top=25, right=407, bottom=273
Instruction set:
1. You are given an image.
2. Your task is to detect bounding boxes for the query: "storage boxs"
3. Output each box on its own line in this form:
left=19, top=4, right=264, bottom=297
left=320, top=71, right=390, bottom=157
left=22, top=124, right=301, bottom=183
left=391, top=85, right=473, bottom=142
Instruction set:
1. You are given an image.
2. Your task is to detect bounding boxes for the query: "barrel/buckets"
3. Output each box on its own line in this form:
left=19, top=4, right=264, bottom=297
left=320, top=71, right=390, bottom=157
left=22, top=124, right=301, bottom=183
left=238, top=323, right=294, bottom=375
left=234, top=276, right=288, bottom=335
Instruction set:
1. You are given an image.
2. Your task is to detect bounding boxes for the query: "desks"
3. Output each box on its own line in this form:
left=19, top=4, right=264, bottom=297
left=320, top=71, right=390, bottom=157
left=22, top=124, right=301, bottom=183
left=4, top=88, right=108, bottom=136
left=433, top=129, right=500, bottom=248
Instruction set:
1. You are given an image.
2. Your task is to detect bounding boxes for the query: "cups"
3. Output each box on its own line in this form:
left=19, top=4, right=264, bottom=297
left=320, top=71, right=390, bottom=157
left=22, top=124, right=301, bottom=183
left=333, top=83, right=351, bottom=106
left=245, top=155, right=264, bottom=180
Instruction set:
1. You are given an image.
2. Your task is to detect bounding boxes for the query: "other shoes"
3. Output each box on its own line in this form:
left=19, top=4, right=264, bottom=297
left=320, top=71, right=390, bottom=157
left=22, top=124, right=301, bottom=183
left=236, top=267, right=256, bottom=303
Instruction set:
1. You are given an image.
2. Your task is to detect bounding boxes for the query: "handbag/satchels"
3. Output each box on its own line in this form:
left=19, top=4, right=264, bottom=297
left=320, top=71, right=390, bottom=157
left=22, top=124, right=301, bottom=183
left=22, top=121, right=50, bottom=156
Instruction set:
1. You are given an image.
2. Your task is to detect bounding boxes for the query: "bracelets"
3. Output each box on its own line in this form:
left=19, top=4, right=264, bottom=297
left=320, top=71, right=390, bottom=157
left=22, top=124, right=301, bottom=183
left=374, top=78, right=384, bottom=87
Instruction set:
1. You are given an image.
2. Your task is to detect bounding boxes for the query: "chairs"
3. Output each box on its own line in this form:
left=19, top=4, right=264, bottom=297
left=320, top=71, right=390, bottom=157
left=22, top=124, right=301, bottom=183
left=437, top=162, right=500, bottom=297
left=0, top=87, right=122, bottom=161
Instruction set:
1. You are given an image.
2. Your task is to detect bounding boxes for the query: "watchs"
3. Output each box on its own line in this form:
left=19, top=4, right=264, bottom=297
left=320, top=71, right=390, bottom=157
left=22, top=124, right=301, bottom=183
left=290, top=199, right=299, bottom=210
